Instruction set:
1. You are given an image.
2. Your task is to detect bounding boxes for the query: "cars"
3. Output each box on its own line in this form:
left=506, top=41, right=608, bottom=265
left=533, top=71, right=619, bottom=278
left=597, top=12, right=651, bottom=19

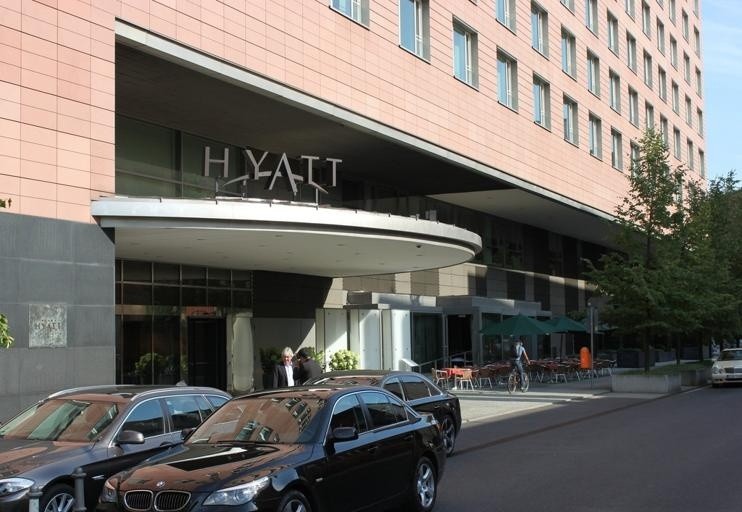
left=711, top=347, right=741, bottom=386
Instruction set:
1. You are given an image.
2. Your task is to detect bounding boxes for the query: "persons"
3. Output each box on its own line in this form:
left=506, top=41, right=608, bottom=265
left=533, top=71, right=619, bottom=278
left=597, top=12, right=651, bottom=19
left=293, top=347, right=323, bottom=384
left=508, top=339, right=532, bottom=389
left=272, top=345, right=296, bottom=389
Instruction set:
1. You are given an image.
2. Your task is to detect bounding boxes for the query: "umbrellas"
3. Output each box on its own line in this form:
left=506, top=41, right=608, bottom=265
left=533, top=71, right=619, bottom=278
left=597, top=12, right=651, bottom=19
left=544, top=312, right=586, bottom=362
left=478, top=311, right=555, bottom=339
left=578, top=316, right=619, bottom=332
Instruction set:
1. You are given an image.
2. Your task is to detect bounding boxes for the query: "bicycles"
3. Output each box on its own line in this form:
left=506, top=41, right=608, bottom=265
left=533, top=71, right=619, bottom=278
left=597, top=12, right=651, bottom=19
left=507, top=367, right=530, bottom=394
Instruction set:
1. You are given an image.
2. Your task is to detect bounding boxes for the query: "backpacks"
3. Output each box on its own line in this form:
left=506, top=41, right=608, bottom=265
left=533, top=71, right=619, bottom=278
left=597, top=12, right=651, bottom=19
left=509, top=343, right=521, bottom=358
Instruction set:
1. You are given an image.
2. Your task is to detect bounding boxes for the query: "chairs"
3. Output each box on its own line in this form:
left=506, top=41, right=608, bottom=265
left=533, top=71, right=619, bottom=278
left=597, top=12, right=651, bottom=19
left=431, top=353, right=617, bottom=392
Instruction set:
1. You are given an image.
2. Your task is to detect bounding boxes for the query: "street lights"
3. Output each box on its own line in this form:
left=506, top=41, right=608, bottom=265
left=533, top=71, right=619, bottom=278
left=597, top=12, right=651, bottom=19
left=587, top=302, right=594, bottom=390
